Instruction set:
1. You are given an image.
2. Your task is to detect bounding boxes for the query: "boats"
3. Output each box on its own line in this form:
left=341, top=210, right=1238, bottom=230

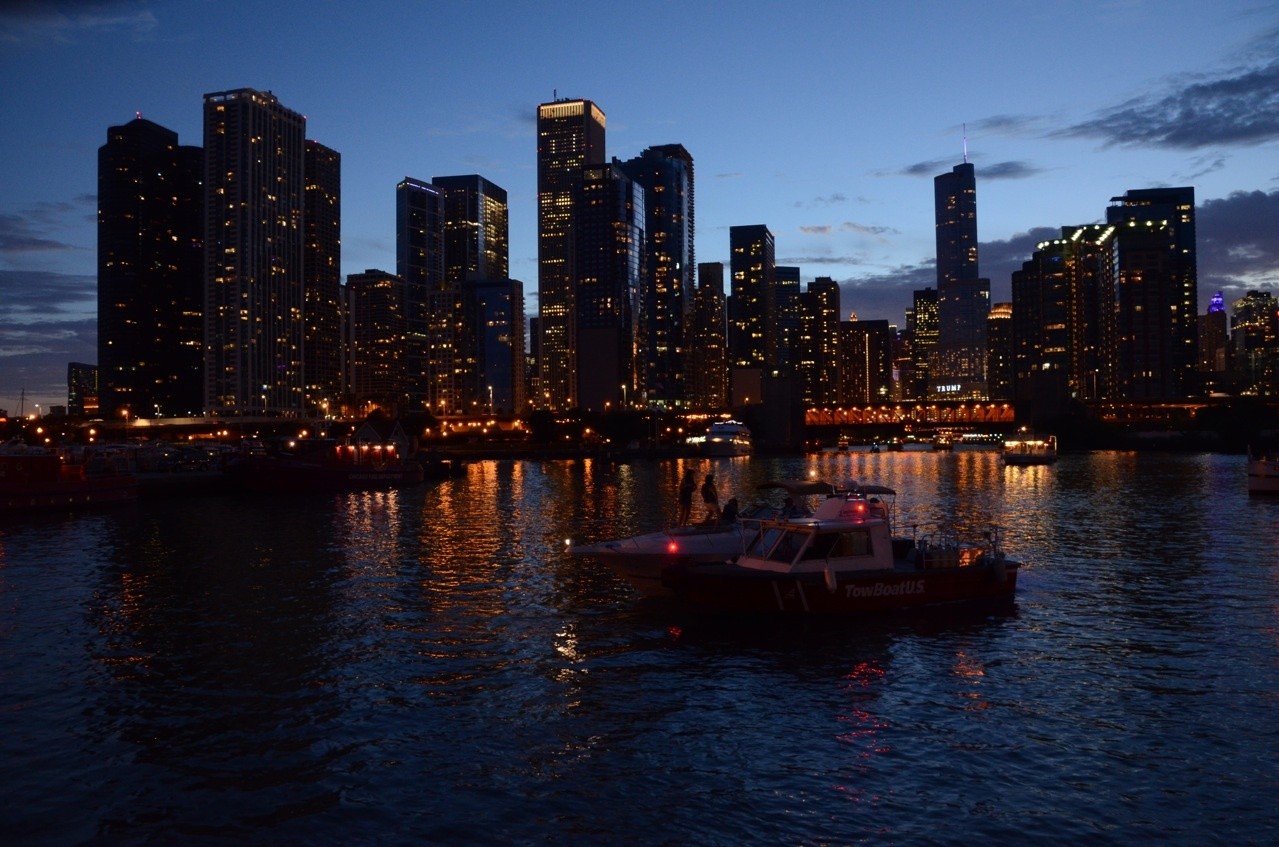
left=887, top=437, right=903, bottom=451
left=1247, top=454, right=1279, bottom=498
left=931, top=435, right=953, bottom=449
left=245, top=423, right=408, bottom=487
left=0, top=453, right=138, bottom=510
left=706, top=418, right=752, bottom=456
left=837, top=437, right=849, bottom=449
left=1002, top=435, right=1059, bottom=464
left=566, top=480, right=1022, bottom=614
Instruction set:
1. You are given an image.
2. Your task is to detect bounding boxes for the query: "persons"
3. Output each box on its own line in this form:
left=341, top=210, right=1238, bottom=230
left=722, top=498, right=742, bottom=526
left=748, top=496, right=797, bottom=517
left=678, top=469, right=697, bottom=529
left=701, top=475, right=721, bottom=532
